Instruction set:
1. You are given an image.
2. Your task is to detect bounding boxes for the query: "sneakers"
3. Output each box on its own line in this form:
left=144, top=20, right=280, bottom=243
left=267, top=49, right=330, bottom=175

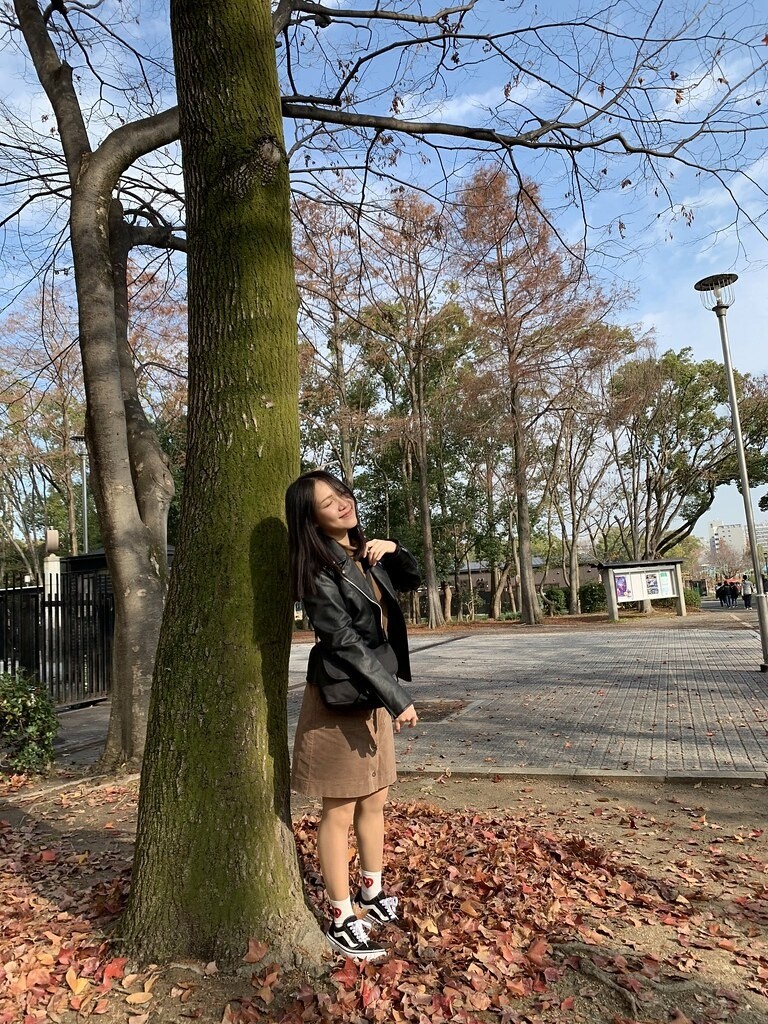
left=352, top=890, right=399, bottom=928
left=325, top=916, right=387, bottom=961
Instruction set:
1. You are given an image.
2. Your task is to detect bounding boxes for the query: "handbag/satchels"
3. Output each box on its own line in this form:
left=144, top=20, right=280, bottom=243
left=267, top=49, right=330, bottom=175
left=310, top=640, right=398, bottom=711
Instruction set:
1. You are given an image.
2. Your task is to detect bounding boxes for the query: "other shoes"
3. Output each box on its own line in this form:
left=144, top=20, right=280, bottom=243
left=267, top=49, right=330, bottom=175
left=727, top=605, right=738, bottom=609
left=744, top=607, right=752, bottom=610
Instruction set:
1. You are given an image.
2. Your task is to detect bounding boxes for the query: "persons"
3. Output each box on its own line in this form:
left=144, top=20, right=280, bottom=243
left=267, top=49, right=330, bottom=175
left=761, top=574, right=768, bottom=605
left=285, top=470, right=422, bottom=960
left=716, top=575, right=754, bottom=611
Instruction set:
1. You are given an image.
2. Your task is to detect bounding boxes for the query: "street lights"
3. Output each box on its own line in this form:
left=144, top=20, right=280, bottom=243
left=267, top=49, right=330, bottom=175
left=23, top=573, right=31, bottom=587
left=694, top=272, right=768, bottom=675
left=763, top=553, right=768, bottom=576
left=71, top=434, right=90, bottom=553
